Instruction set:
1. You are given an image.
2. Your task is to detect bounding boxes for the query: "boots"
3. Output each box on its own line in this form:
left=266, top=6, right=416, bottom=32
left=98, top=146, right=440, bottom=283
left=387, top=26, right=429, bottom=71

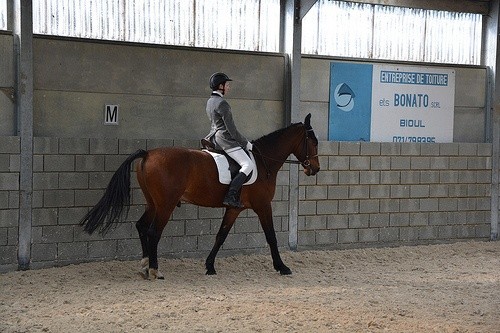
left=222, top=172, right=246, bottom=207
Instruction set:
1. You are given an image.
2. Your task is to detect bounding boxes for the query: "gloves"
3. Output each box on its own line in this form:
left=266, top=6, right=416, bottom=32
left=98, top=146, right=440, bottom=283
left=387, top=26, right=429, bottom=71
left=246, top=141, right=253, bottom=151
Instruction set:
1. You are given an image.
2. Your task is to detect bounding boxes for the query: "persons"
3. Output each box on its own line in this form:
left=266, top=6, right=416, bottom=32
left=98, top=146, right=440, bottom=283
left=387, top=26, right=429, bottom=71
left=205, top=72, right=254, bottom=208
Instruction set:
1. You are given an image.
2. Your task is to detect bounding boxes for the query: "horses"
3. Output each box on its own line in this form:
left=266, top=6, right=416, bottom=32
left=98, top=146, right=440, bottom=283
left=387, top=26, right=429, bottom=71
left=78, top=112, right=321, bottom=280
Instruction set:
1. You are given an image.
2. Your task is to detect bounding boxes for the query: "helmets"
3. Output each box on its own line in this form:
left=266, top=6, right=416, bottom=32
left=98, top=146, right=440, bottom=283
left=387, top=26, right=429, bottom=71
left=209, top=72, right=233, bottom=88
